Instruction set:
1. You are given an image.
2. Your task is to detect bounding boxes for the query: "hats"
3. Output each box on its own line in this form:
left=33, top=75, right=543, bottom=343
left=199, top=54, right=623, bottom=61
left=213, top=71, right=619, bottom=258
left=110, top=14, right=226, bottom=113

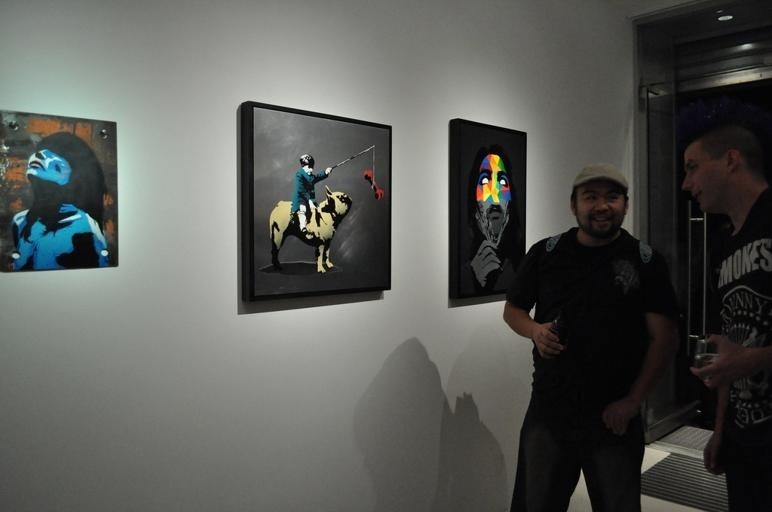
left=572, top=163, right=629, bottom=189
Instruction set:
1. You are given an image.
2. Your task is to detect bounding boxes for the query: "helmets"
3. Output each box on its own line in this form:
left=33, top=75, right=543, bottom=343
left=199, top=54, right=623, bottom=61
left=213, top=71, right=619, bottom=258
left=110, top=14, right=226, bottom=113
left=300, top=154, right=315, bottom=166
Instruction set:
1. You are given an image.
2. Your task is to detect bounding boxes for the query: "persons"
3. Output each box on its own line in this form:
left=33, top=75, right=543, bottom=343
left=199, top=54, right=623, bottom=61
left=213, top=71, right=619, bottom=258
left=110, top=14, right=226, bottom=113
left=10, top=132, right=110, bottom=268
left=293, top=154, right=331, bottom=240
left=680, top=125, right=771, bottom=512
left=448, top=146, right=513, bottom=297
left=503, top=162, right=674, bottom=512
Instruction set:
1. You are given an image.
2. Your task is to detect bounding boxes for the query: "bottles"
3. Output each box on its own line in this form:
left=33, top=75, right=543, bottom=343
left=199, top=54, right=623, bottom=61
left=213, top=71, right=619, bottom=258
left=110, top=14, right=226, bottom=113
left=548, top=305, right=568, bottom=338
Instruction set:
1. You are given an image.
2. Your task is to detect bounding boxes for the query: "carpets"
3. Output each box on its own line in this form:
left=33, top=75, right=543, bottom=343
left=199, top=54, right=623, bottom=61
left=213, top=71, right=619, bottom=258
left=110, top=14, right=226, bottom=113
left=641, top=453, right=731, bottom=511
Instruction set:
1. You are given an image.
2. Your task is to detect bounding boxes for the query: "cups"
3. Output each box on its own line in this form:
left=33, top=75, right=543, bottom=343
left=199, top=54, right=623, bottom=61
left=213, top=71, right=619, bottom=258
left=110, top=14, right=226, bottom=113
left=696, top=340, right=723, bottom=380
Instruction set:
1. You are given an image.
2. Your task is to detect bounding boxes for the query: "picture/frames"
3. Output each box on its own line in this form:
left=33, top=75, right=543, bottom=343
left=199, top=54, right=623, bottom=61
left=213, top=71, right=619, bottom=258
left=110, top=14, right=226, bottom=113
left=239, top=101, right=393, bottom=302
